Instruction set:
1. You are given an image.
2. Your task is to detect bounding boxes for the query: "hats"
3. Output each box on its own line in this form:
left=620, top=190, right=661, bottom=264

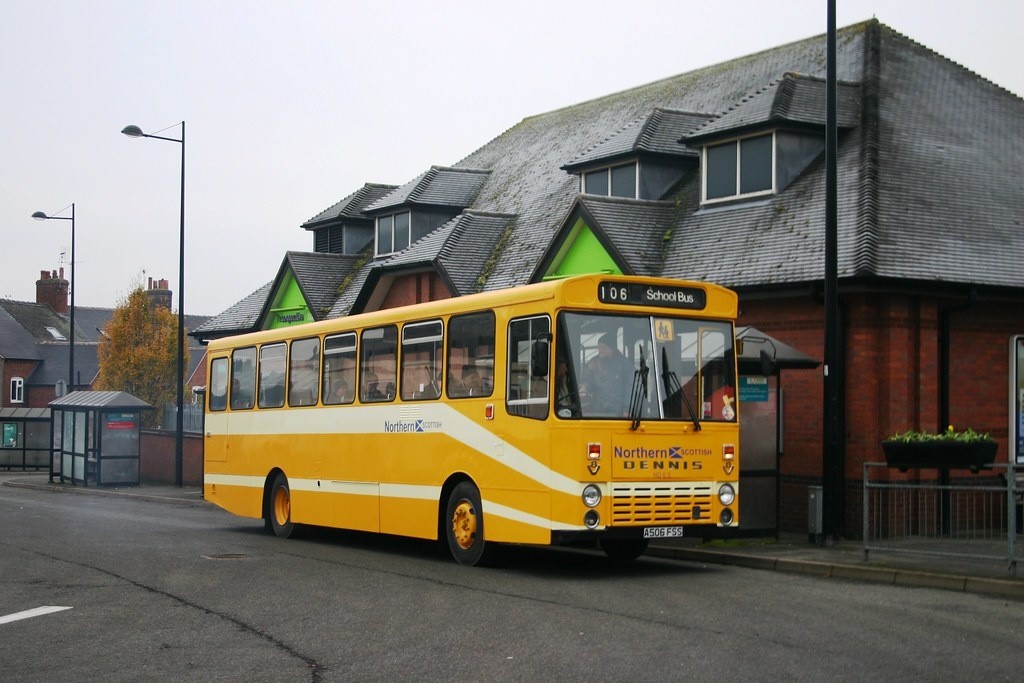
left=437, top=370, right=454, bottom=384
left=597, top=333, right=617, bottom=350
left=367, top=373, right=378, bottom=382
left=333, top=378, right=349, bottom=392
left=461, top=364, right=478, bottom=380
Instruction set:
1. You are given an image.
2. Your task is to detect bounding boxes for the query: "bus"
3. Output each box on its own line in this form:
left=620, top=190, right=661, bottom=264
left=203, top=274, right=741, bottom=567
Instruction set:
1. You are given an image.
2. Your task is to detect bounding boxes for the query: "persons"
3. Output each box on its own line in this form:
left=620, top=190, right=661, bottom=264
left=586, top=333, right=636, bottom=414
left=362, top=374, right=384, bottom=401
left=451, top=364, right=481, bottom=393
left=326, top=381, right=352, bottom=403
left=223, top=378, right=240, bottom=397
left=421, top=373, right=453, bottom=398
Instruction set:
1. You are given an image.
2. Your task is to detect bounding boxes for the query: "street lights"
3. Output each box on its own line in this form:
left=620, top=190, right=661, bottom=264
left=31, top=202, right=76, bottom=394
left=120, top=119, right=186, bottom=488
